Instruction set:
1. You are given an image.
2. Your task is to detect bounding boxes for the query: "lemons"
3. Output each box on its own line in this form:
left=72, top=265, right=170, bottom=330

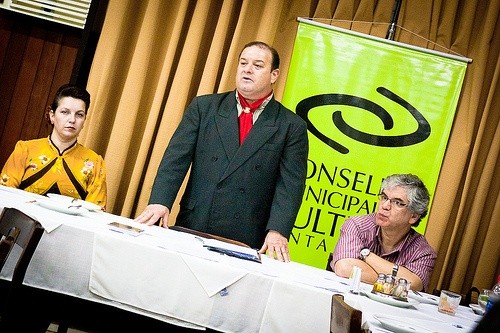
left=478, top=295, right=489, bottom=302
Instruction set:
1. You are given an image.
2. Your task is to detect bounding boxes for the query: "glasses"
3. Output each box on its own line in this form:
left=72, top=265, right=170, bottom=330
left=377, top=193, right=414, bottom=210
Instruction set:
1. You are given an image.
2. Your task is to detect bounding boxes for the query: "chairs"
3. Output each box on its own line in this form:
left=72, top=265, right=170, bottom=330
left=465, top=287, right=480, bottom=308
left=0, top=205, right=46, bottom=333
left=168, top=226, right=252, bottom=249
left=326, top=252, right=334, bottom=273
left=329, top=292, right=363, bottom=333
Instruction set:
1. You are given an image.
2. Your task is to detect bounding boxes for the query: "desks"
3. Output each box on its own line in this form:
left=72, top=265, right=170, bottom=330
left=0, top=183, right=484, bottom=333
left=357, top=306, right=473, bottom=333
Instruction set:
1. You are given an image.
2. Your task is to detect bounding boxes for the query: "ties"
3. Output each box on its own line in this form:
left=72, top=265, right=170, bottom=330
left=238, top=89, right=273, bottom=145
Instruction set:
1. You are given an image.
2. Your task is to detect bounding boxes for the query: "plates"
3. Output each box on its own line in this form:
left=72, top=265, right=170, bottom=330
left=407, top=290, right=440, bottom=304
left=35, top=196, right=90, bottom=216
left=47, top=193, right=101, bottom=211
left=371, top=313, right=438, bottom=333
left=362, top=287, right=414, bottom=308
left=469, top=303, right=485, bottom=315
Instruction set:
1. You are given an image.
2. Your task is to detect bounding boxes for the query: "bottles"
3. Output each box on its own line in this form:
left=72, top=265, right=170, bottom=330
left=371, top=273, right=412, bottom=301
left=492, top=276, right=500, bottom=300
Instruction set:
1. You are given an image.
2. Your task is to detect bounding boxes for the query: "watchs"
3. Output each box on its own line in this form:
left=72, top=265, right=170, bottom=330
left=361, top=248, right=371, bottom=262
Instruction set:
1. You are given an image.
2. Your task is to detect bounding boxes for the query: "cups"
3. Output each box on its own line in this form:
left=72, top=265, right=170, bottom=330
left=478, top=288, right=492, bottom=308
left=438, top=289, right=462, bottom=315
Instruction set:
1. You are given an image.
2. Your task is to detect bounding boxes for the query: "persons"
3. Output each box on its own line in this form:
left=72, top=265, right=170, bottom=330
left=329, top=174, right=437, bottom=293
left=133, top=42, right=309, bottom=264
left=0, top=82, right=107, bottom=211
